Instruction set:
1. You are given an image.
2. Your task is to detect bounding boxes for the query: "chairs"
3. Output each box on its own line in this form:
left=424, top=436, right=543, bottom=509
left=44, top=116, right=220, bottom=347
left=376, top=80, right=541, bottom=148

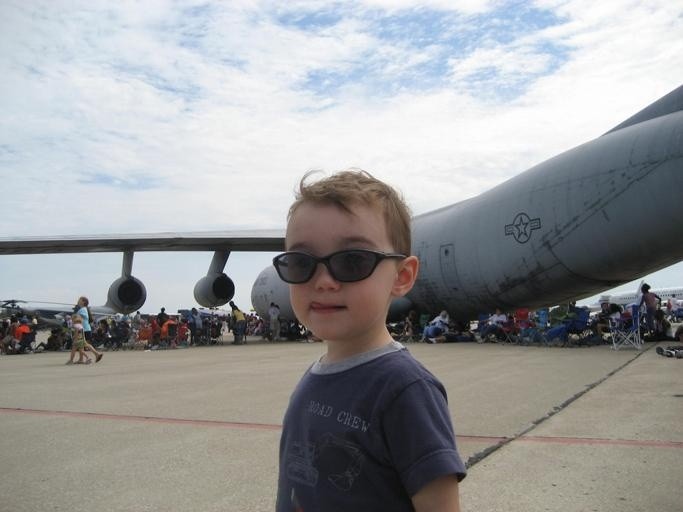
left=425, top=314, right=452, bottom=343
left=8, top=331, right=37, bottom=354
left=112, top=320, right=223, bottom=351
left=478, top=305, right=641, bottom=351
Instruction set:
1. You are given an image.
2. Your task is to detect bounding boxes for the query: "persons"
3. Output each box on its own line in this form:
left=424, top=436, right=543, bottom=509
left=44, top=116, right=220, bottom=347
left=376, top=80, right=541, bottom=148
left=1, top=301, right=320, bottom=353
left=66, top=296, right=103, bottom=363
left=271, top=164, right=468, bottom=512
left=656, top=346, right=683, bottom=358
left=470, top=282, right=683, bottom=341
left=388, top=310, right=469, bottom=343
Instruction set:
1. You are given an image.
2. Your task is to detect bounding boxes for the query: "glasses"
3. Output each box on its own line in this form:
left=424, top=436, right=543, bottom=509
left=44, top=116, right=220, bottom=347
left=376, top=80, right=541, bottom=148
left=272, top=248, right=407, bottom=285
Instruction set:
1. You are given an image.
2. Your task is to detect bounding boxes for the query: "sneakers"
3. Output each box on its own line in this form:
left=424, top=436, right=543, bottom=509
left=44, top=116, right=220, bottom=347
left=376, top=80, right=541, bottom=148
left=654, top=346, right=679, bottom=357
left=65, top=352, right=103, bottom=366
